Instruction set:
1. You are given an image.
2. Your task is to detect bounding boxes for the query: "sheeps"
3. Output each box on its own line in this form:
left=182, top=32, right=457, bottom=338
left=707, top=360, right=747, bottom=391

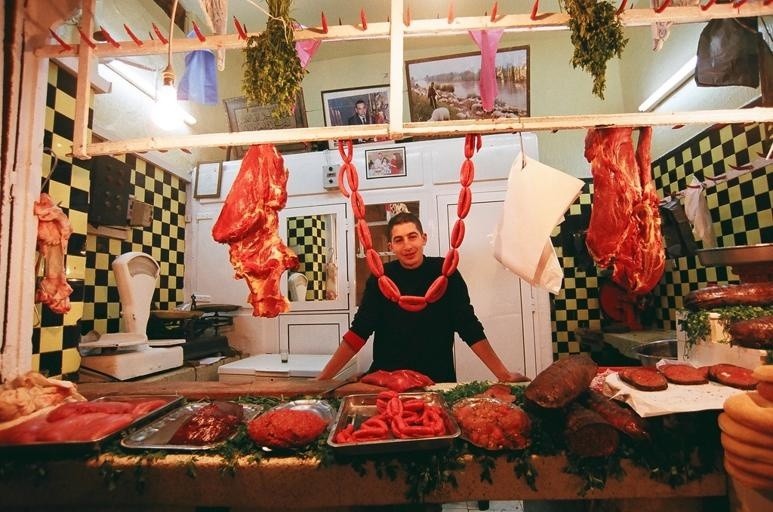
left=410, top=84, right=529, bottom=122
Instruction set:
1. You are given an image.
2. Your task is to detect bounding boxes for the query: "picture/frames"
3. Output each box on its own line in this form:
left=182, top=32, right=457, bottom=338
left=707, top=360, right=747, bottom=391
left=364, top=147, right=408, bottom=179
left=321, top=84, right=399, bottom=149
left=404, top=45, right=531, bottom=122
left=225, top=89, right=311, bottom=160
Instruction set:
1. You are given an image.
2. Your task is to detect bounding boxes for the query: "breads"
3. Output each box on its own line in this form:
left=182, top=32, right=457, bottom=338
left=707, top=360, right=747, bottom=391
left=719, top=365, right=773, bottom=488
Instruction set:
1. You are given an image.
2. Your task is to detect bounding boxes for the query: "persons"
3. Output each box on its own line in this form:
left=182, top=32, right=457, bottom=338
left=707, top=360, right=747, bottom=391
left=373, top=152, right=382, bottom=169
left=313, top=211, right=528, bottom=386
left=427, top=81, right=437, bottom=110
left=347, top=102, right=373, bottom=144
left=379, top=158, right=397, bottom=168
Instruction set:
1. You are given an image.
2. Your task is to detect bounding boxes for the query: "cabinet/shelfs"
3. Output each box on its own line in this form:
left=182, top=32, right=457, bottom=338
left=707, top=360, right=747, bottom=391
left=346, top=199, right=436, bottom=310
left=440, top=191, right=534, bottom=380
left=278, top=203, right=350, bottom=356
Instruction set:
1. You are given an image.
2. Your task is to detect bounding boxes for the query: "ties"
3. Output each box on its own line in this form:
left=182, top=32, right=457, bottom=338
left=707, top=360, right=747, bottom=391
left=363, top=118, right=366, bottom=124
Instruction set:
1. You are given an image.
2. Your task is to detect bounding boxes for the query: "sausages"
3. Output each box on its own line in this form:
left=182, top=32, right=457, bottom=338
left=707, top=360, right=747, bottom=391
left=335, top=391, right=446, bottom=443
left=338, top=136, right=482, bottom=311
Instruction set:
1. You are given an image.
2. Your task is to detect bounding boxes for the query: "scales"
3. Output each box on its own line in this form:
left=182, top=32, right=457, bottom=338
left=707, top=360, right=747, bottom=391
left=79, top=252, right=186, bottom=380
left=151, top=294, right=242, bottom=362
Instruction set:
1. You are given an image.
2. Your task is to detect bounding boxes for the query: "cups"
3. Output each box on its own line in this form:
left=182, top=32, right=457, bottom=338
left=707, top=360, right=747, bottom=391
left=281, top=351, right=288, bottom=363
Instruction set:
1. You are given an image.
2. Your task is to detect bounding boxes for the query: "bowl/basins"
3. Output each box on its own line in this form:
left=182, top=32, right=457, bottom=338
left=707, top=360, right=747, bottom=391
left=631, top=338, right=678, bottom=369
